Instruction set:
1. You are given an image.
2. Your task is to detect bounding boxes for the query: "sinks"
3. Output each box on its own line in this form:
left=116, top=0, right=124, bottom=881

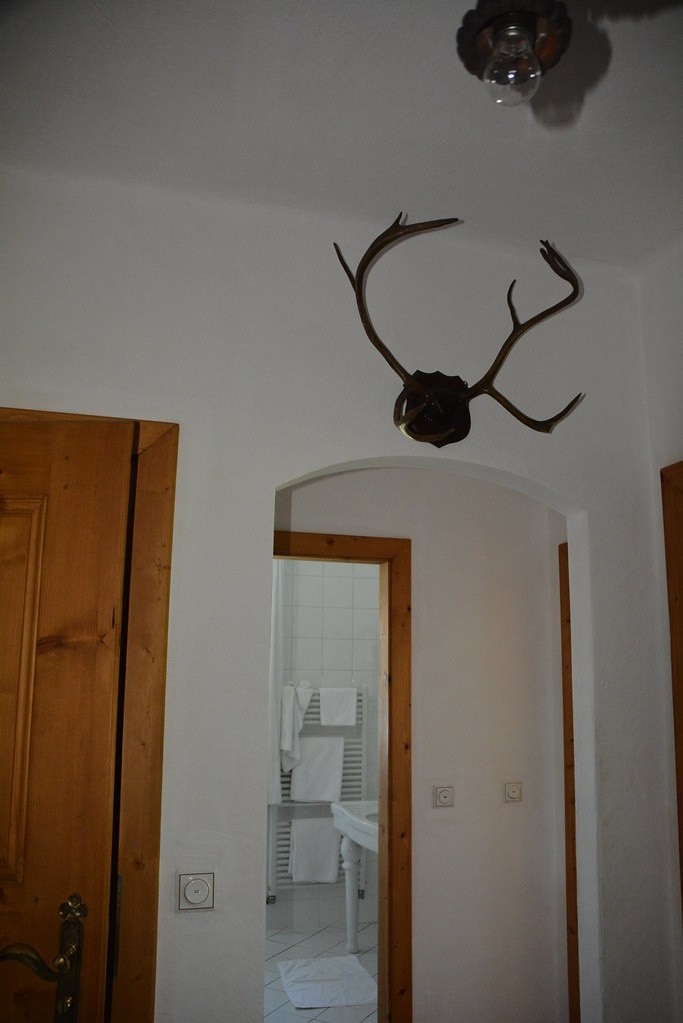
left=330, top=799, right=379, bottom=855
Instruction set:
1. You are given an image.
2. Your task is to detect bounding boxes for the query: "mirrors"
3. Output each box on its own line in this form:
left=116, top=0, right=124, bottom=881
left=260, top=455, right=580, bottom=1023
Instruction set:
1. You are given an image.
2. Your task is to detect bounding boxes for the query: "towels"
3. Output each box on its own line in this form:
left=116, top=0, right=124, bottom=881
left=290, top=735, right=345, bottom=803
left=318, top=687, right=358, bottom=728
left=278, top=686, right=314, bottom=773
left=287, top=816, right=341, bottom=884
